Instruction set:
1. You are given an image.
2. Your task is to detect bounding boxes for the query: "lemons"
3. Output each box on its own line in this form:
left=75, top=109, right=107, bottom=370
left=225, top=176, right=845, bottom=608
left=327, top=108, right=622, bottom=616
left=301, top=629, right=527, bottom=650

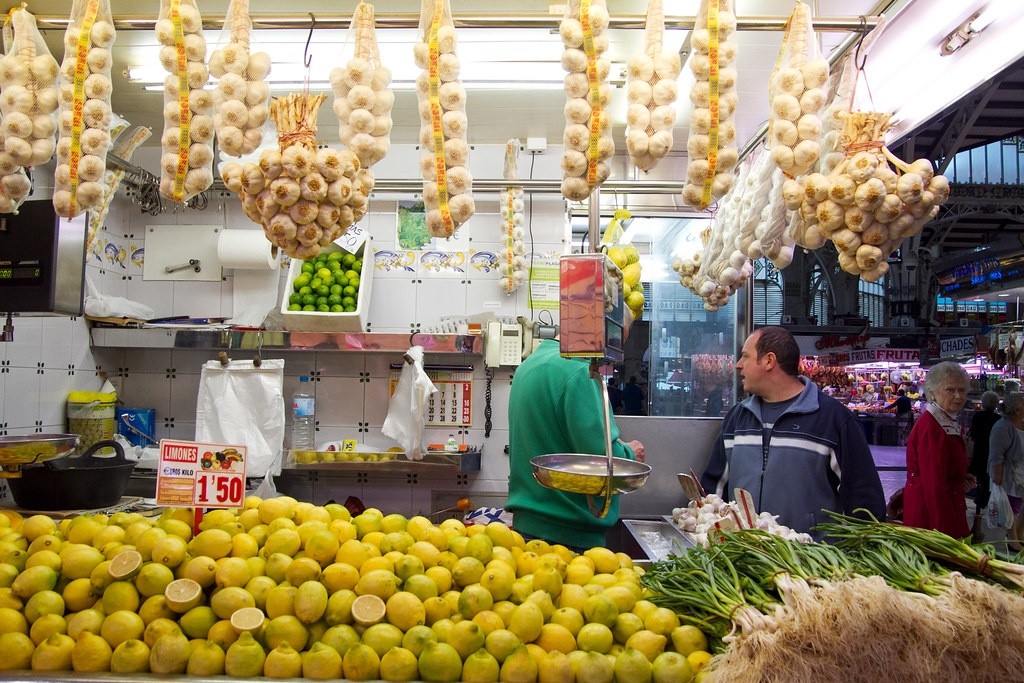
left=288, top=252, right=363, bottom=312
left=604, top=244, right=645, bottom=321
left=298, top=453, right=391, bottom=465
left=0, top=496, right=718, bottom=683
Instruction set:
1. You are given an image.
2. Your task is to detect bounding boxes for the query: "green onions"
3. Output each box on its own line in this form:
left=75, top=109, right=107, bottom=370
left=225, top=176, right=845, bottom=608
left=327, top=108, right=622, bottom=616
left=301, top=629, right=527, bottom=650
left=636, top=505, right=1024, bottom=683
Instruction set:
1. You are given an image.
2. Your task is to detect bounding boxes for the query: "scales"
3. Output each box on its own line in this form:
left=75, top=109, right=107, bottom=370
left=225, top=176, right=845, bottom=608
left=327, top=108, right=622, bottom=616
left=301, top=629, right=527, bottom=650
left=529, top=252, right=653, bottom=519
left=0, top=200, right=82, bottom=479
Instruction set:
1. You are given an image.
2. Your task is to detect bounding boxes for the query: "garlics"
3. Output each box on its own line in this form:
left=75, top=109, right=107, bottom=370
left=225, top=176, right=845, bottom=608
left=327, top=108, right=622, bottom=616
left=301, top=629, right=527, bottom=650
left=672, top=494, right=813, bottom=548
left=0, top=0, right=616, bottom=293
left=624, top=8, right=951, bottom=311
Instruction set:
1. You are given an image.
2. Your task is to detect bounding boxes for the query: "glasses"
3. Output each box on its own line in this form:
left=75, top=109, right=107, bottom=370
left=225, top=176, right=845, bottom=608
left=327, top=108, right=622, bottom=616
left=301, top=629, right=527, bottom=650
left=939, top=387, right=967, bottom=394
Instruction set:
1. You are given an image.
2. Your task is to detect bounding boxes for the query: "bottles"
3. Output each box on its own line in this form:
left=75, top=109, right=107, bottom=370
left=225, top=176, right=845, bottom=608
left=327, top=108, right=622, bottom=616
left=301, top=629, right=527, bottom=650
left=445, top=434, right=458, bottom=451
left=291, top=375, right=316, bottom=450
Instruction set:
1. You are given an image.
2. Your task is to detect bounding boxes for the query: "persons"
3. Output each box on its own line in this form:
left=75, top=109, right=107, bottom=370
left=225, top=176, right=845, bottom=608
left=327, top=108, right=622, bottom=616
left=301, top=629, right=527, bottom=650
left=988, top=393, right=1024, bottom=552
left=700, top=326, right=886, bottom=547
left=607, top=375, right=644, bottom=416
left=504, top=335, right=646, bottom=555
left=904, top=361, right=971, bottom=540
left=967, top=391, right=1002, bottom=513
left=883, top=389, right=911, bottom=418
left=705, top=382, right=724, bottom=418
left=854, top=384, right=886, bottom=406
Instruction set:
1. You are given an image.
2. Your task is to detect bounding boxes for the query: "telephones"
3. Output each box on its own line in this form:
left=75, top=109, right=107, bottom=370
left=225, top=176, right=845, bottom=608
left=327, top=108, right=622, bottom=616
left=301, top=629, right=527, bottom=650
left=486, top=322, right=522, bottom=366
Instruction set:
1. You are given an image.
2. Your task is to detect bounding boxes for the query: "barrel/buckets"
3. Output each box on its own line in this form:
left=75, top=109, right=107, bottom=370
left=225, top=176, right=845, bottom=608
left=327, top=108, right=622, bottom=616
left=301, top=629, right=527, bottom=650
left=4, top=439, right=139, bottom=510
left=68, top=401, right=115, bottom=454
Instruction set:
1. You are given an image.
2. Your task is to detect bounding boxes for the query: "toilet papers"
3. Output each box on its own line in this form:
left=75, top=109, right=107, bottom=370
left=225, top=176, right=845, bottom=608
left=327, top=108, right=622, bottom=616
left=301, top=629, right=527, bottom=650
left=217, top=229, right=281, bottom=327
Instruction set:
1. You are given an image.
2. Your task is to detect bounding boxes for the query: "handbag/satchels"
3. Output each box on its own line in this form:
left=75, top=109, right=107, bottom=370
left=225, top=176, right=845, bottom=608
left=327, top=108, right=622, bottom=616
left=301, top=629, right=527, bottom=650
left=987, top=482, right=1014, bottom=530
left=886, top=488, right=905, bottom=519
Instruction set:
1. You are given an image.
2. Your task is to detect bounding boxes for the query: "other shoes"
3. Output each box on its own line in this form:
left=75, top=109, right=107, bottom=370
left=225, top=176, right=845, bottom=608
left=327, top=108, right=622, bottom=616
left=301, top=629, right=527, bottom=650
left=1008, top=544, right=1021, bottom=554
left=1019, top=542, right=1024, bottom=546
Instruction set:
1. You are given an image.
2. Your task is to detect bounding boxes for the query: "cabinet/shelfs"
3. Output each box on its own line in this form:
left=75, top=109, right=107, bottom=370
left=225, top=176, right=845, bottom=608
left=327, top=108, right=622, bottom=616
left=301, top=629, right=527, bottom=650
left=90, top=329, right=485, bottom=474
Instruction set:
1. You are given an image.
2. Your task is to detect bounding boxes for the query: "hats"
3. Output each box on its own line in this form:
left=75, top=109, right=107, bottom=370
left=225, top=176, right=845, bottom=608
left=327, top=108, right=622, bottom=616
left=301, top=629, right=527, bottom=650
left=1003, top=393, right=1023, bottom=412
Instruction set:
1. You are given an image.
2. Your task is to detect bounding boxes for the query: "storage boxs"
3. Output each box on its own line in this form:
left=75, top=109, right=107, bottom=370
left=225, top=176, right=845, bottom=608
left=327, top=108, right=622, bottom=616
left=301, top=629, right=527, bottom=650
left=281, top=236, right=374, bottom=332
left=118, top=407, right=157, bottom=448
